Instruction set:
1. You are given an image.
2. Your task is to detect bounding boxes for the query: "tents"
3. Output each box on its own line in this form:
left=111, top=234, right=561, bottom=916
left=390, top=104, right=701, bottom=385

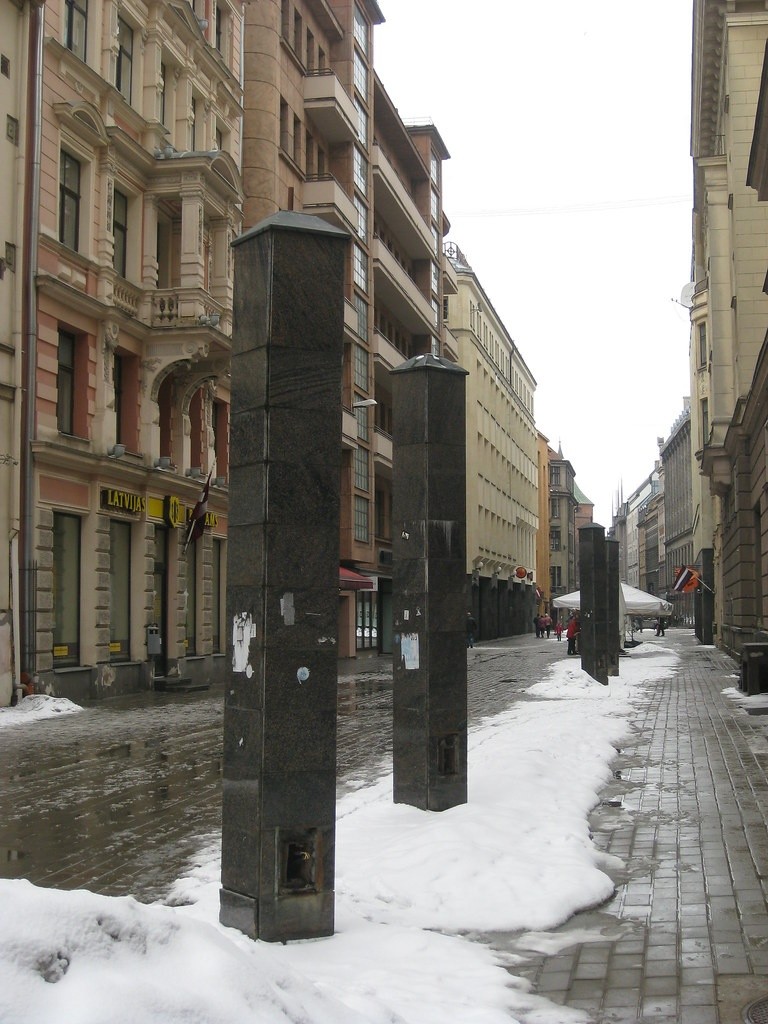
left=553, top=582, right=674, bottom=648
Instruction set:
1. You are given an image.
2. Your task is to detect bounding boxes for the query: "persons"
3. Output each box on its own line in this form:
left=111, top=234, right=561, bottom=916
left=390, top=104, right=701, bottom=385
left=466, top=612, right=477, bottom=648
left=654, top=615, right=665, bottom=636
left=566, top=613, right=578, bottom=655
left=534, top=613, right=552, bottom=638
left=555, top=621, right=563, bottom=641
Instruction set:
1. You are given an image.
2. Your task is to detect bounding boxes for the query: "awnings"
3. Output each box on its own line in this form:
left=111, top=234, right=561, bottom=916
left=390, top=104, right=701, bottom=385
left=339, top=567, right=373, bottom=593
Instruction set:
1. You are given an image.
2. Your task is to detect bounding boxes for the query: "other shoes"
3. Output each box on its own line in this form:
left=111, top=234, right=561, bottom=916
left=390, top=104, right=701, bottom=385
left=655, top=635, right=659, bottom=636
left=467, top=644, right=469, bottom=648
left=470, top=645, right=473, bottom=649
left=573, top=652, right=580, bottom=655
left=661, top=635, right=664, bottom=636
left=568, top=653, right=574, bottom=655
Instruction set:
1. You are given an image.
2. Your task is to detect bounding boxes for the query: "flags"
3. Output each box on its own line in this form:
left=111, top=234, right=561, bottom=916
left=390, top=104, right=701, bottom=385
left=672, top=565, right=693, bottom=591
left=188, top=473, right=212, bottom=544
